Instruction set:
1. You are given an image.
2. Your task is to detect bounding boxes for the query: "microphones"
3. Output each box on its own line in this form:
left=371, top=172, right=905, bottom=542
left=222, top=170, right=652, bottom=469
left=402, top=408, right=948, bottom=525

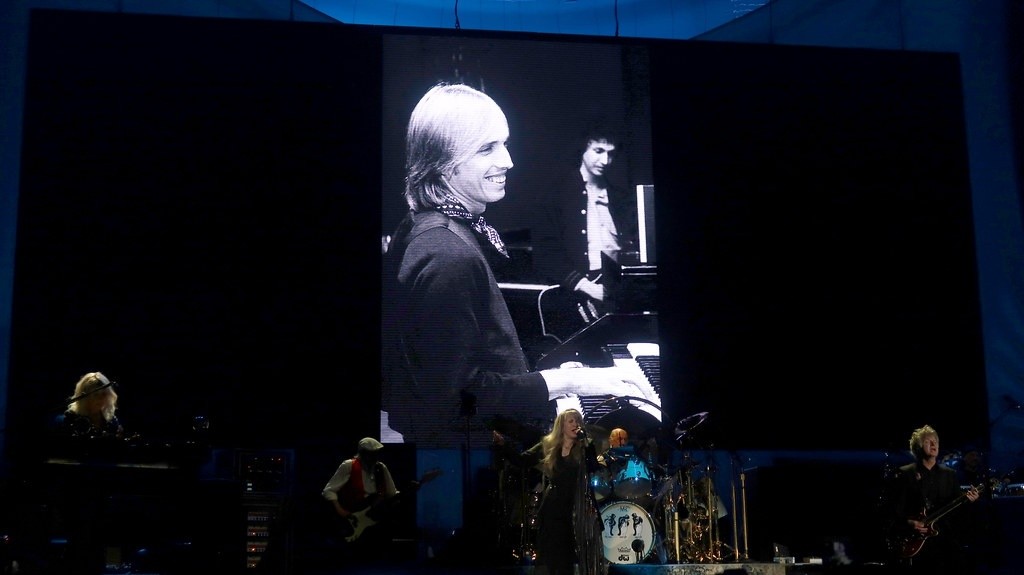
left=576, top=431, right=585, bottom=439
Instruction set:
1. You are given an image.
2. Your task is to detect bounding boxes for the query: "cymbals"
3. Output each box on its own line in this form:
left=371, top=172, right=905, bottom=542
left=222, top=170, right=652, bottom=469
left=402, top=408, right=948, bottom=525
left=675, top=409, right=708, bottom=434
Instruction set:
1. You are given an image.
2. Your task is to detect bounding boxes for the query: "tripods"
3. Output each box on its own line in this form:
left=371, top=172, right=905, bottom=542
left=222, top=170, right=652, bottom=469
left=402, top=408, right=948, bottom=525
left=653, top=469, right=745, bottom=563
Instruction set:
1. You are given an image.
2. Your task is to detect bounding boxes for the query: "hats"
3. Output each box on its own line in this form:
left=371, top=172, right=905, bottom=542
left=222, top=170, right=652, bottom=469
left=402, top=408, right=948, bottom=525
left=359, top=437, right=384, bottom=451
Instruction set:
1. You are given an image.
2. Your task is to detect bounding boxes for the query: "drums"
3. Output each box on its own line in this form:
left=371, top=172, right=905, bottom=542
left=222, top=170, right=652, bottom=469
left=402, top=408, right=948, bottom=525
left=599, top=500, right=657, bottom=565
left=612, top=454, right=652, bottom=499
left=588, top=465, right=612, bottom=503
left=660, top=464, right=728, bottom=533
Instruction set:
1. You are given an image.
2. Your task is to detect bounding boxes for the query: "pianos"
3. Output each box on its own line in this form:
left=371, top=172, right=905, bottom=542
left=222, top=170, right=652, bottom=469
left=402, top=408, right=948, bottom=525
left=534, top=248, right=662, bottom=430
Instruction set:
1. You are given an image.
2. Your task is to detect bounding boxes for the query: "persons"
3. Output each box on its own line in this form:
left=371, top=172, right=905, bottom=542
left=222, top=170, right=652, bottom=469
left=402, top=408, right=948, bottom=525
left=320, top=437, right=399, bottom=519
left=892, top=424, right=980, bottom=575
left=64, top=369, right=126, bottom=436
left=492, top=408, right=629, bottom=574
left=385, top=83, right=654, bottom=450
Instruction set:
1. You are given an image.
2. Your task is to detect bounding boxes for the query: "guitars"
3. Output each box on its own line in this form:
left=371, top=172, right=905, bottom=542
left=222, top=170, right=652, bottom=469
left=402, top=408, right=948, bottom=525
left=345, top=466, right=441, bottom=543
left=888, top=481, right=985, bottom=559
